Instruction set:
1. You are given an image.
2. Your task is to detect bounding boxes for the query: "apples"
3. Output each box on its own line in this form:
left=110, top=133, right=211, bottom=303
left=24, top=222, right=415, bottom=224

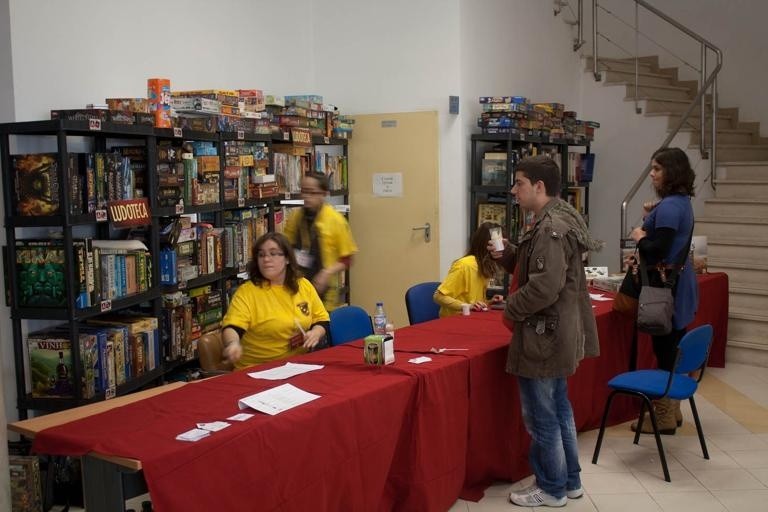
left=492, top=294, right=503, bottom=304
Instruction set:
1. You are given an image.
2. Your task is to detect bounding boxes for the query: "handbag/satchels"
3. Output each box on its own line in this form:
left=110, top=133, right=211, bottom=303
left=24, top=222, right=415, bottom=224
left=613, top=265, right=641, bottom=317
left=636, top=285, right=674, bottom=335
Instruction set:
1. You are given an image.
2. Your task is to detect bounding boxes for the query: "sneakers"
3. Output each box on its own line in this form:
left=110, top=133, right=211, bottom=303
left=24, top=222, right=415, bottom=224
left=509, top=482, right=567, bottom=506
left=565, top=486, right=583, bottom=499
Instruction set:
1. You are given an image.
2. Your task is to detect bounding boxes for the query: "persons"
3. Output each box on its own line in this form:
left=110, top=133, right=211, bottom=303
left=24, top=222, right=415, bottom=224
left=630, top=149, right=696, bottom=435
left=434, top=221, right=504, bottom=319
left=487, top=155, right=601, bottom=507
left=221, top=230, right=330, bottom=372
left=282, top=167, right=359, bottom=313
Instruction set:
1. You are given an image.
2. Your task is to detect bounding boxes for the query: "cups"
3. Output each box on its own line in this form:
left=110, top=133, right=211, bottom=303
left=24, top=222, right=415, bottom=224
left=487, top=227, right=505, bottom=251
left=386, top=321, right=394, bottom=342
left=460, top=303, right=470, bottom=317
left=368, top=341, right=378, bottom=364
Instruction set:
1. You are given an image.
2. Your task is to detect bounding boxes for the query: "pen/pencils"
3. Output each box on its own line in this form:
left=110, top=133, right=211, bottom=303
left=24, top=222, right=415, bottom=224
left=294, top=318, right=305, bottom=336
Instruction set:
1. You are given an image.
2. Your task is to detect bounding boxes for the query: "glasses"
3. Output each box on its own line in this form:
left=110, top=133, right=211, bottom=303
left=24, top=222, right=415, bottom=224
left=257, top=251, right=285, bottom=257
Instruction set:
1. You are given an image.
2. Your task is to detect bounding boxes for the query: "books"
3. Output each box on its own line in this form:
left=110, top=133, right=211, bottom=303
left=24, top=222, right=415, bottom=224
left=480, top=94, right=601, bottom=145
left=10, top=84, right=355, bottom=386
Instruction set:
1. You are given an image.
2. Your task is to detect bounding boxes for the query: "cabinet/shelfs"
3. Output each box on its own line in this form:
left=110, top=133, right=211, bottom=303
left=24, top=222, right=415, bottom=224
left=1, top=114, right=352, bottom=508
left=469, top=133, right=591, bottom=299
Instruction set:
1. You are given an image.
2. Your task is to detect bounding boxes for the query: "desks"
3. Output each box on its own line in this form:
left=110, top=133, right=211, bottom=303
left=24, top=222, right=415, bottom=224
left=343, top=267, right=728, bottom=504
left=4, top=344, right=470, bottom=512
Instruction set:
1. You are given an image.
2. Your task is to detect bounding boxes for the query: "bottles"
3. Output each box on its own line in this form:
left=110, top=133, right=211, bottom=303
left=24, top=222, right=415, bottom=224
left=372, top=302, right=386, bottom=335
left=56, top=351, right=68, bottom=395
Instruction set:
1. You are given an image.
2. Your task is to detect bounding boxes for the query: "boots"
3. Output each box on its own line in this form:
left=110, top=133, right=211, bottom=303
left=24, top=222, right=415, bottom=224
left=631, top=398, right=677, bottom=435
left=675, top=399, right=682, bottom=427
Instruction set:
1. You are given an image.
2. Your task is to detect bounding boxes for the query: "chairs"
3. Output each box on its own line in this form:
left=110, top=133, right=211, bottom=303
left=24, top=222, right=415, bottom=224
left=590, top=324, right=715, bottom=483
left=404, top=281, right=445, bottom=325
left=325, top=304, right=374, bottom=348
